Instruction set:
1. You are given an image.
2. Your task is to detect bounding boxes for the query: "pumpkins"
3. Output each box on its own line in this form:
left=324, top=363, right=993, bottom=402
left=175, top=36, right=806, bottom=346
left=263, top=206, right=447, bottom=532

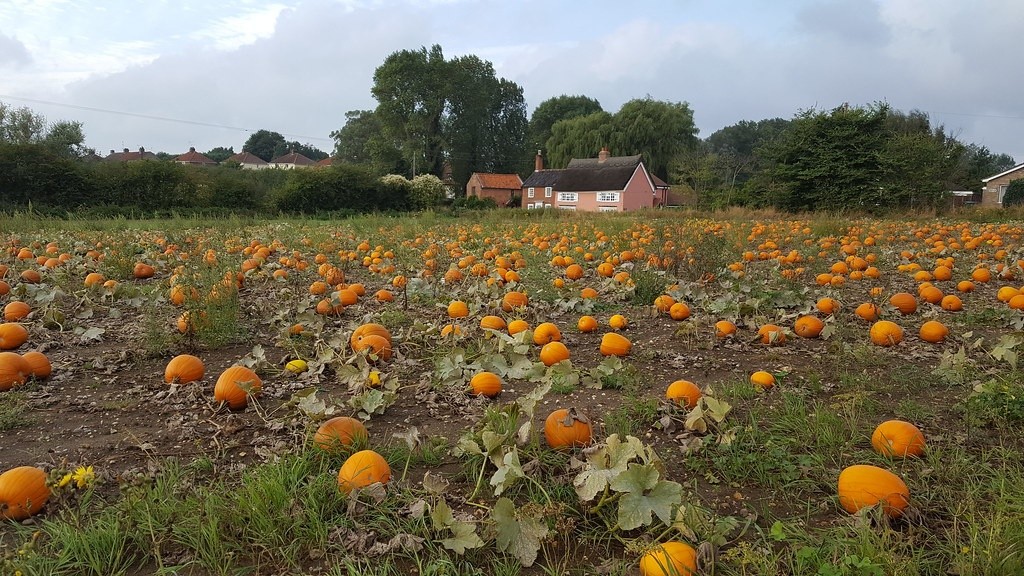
left=0, top=221, right=1024, bottom=576
